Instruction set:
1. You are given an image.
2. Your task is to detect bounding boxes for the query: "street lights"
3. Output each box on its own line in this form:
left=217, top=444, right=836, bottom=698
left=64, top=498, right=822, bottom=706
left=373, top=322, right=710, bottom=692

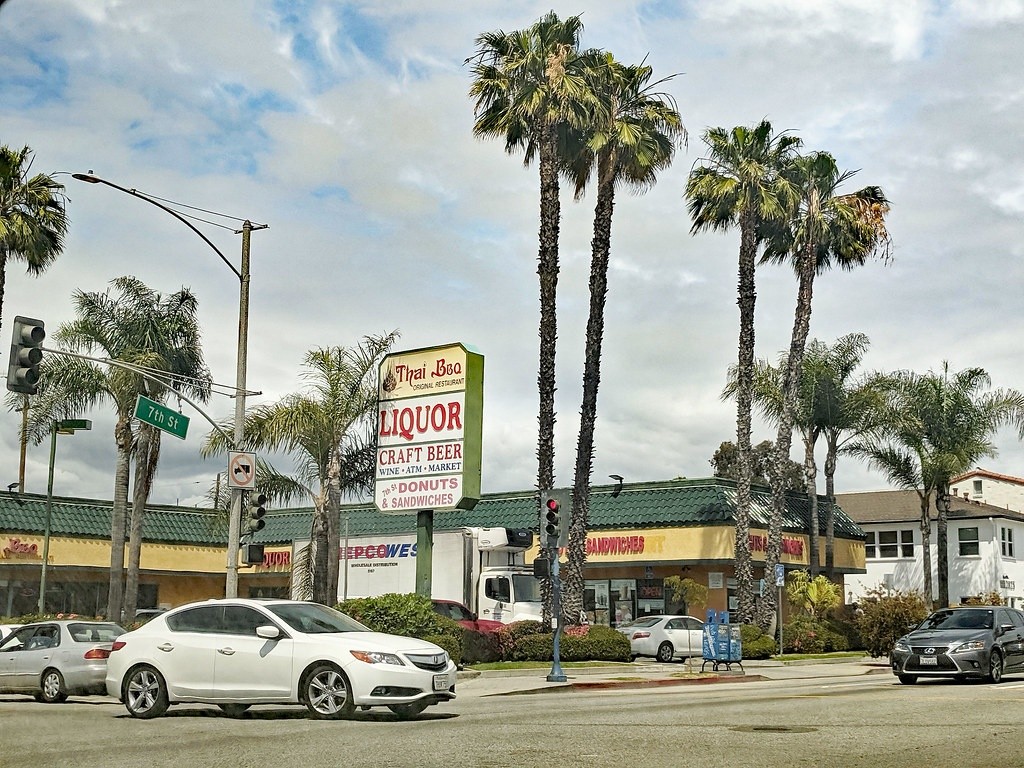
left=39, top=419, right=92, bottom=615
left=72, top=169, right=252, bottom=598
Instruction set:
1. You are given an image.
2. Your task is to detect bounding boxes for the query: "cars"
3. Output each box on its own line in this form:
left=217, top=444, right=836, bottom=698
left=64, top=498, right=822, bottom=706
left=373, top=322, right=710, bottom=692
left=0, top=620, right=128, bottom=703
left=615, top=615, right=705, bottom=663
left=431, top=599, right=513, bottom=653
left=105, top=599, right=457, bottom=720
left=891, top=605, right=1024, bottom=685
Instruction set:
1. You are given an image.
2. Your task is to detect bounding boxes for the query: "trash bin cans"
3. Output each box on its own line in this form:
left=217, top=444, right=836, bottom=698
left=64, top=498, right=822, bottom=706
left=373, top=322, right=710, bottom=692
left=699, top=622, right=744, bottom=673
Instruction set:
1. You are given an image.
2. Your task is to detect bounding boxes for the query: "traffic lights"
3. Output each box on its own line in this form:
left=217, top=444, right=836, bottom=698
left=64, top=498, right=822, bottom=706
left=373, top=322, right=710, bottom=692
left=248, top=492, right=266, bottom=532
left=545, top=499, right=560, bottom=535
left=16, top=323, right=46, bottom=387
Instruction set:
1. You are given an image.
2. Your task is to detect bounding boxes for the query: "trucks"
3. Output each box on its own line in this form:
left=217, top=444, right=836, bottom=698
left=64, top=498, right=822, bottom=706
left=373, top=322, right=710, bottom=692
left=291, top=527, right=589, bottom=627
